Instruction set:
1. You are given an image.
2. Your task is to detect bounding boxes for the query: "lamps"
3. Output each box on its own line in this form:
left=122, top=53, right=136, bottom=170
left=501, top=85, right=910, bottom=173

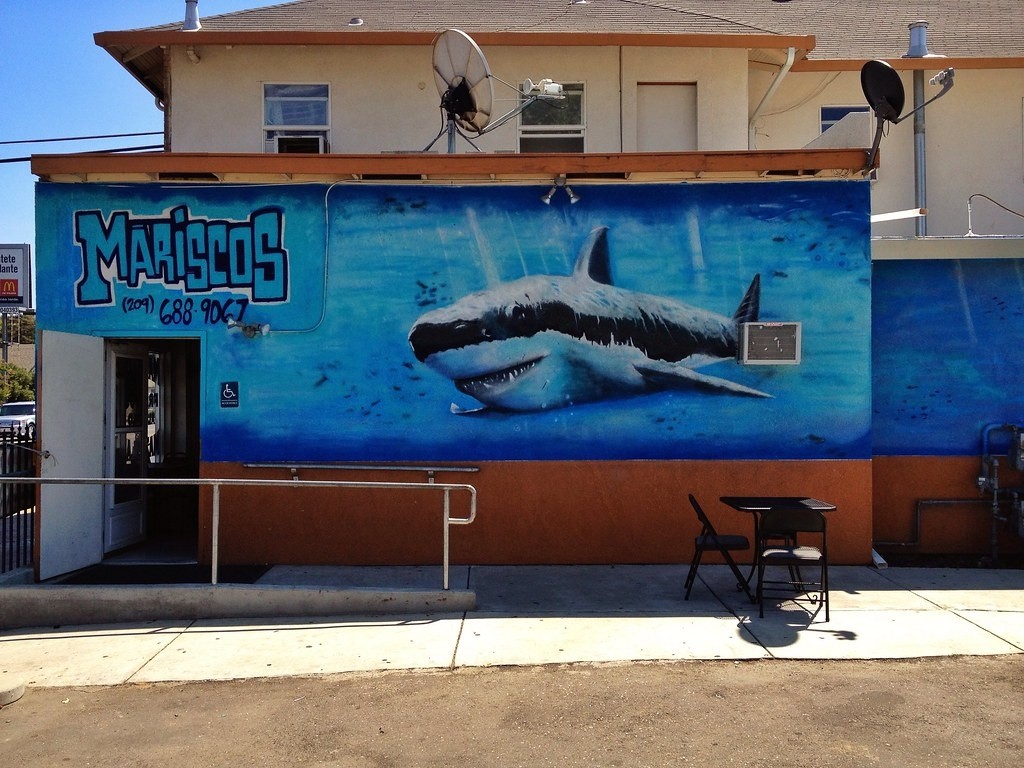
left=252, top=323, right=270, bottom=336
left=565, top=187, right=581, bottom=204
left=227, top=316, right=246, bottom=329
left=539, top=188, right=556, bottom=205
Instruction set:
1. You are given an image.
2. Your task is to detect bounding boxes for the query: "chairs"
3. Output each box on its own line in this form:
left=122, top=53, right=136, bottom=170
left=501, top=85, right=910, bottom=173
left=757, top=507, right=830, bottom=622
left=684, top=494, right=755, bottom=602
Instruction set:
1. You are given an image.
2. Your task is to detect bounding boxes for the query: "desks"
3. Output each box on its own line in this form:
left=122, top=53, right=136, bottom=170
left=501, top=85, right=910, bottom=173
left=719, top=497, right=837, bottom=600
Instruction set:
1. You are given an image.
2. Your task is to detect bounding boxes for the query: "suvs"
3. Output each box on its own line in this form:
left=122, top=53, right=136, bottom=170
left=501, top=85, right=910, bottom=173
left=0, top=401, right=36, bottom=440
